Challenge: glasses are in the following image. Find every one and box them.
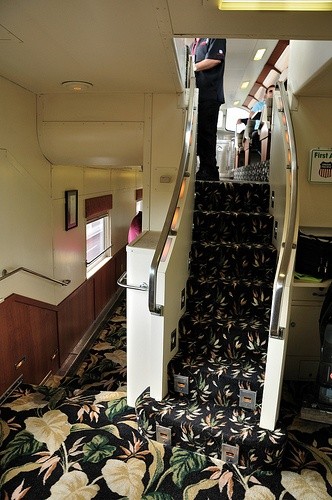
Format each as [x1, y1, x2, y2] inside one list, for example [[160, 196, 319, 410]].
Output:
[[267, 90, 272, 93]]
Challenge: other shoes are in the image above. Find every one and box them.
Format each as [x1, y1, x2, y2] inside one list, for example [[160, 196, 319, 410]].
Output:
[[196, 167, 219, 181]]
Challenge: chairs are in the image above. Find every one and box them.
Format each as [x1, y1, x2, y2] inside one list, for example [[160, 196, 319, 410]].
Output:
[[235, 99, 272, 169]]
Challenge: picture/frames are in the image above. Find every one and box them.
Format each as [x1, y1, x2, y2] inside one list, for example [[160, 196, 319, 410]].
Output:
[[64, 189, 80, 231]]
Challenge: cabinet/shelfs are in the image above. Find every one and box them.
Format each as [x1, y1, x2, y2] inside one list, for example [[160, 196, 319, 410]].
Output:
[[287, 280, 331, 381]]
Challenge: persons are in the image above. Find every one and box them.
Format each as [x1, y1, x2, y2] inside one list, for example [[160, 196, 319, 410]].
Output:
[[265, 85, 276, 101], [189, 37, 226, 181]]
